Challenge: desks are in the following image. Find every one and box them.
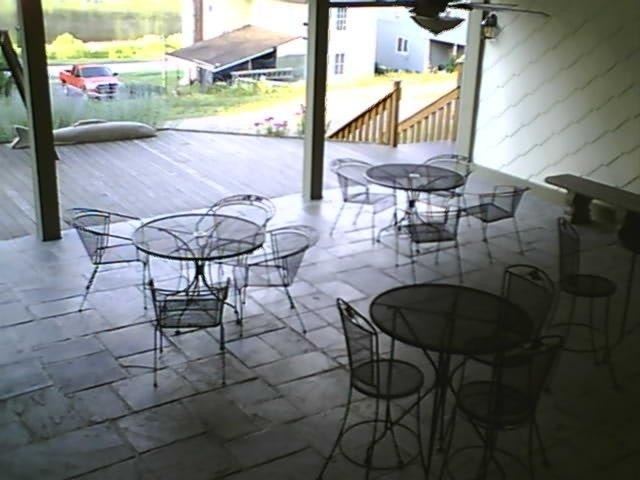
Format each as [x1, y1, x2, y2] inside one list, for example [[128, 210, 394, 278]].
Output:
[[132, 212, 266, 337], [370, 282, 536, 468], [366, 164, 465, 241]]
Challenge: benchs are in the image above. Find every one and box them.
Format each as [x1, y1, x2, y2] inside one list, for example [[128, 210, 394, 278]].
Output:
[[544, 173, 640, 252]]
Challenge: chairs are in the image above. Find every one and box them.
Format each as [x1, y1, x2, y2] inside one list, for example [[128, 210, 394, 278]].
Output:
[[318, 297, 430, 480], [194, 193, 272, 305], [416, 153, 475, 230], [235, 224, 319, 337], [620, 213, 640, 340], [545, 216, 620, 390], [454, 185, 531, 266], [330, 158, 401, 243], [396, 192, 462, 286], [465, 265, 556, 414], [63, 206, 155, 314], [147, 275, 230, 386], [442, 335, 565, 480]]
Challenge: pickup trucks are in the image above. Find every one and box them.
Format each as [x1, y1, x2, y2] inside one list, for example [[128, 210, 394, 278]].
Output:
[[59, 64, 123, 102]]
